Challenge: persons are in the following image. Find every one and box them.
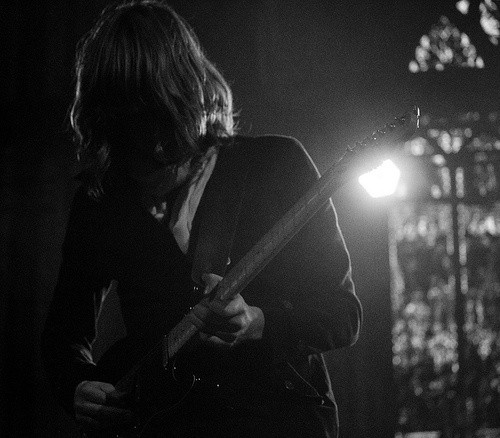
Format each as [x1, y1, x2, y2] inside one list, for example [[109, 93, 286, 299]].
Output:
[[40, 0, 364, 438]]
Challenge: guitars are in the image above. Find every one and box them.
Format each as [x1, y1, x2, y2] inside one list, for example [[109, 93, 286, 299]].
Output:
[[96, 104, 420, 438]]
